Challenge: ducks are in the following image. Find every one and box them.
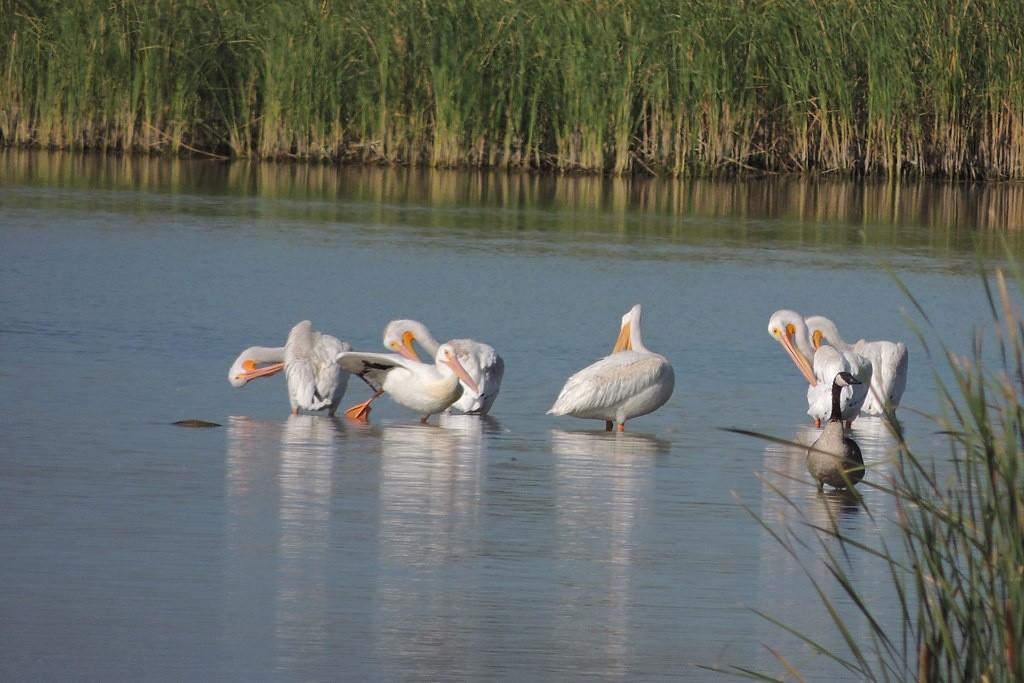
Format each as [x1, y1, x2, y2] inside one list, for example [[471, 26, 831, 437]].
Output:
[[806, 371, 865, 493]]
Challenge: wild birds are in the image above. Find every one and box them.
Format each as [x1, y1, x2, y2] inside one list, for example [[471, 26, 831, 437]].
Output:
[[335, 343, 479, 427], [228, 319, 354, 418], [805, 315, 909, 416], [767, 310, 873, 429], [380, 319, 506, 416], [544, 303, 676, 433]]
